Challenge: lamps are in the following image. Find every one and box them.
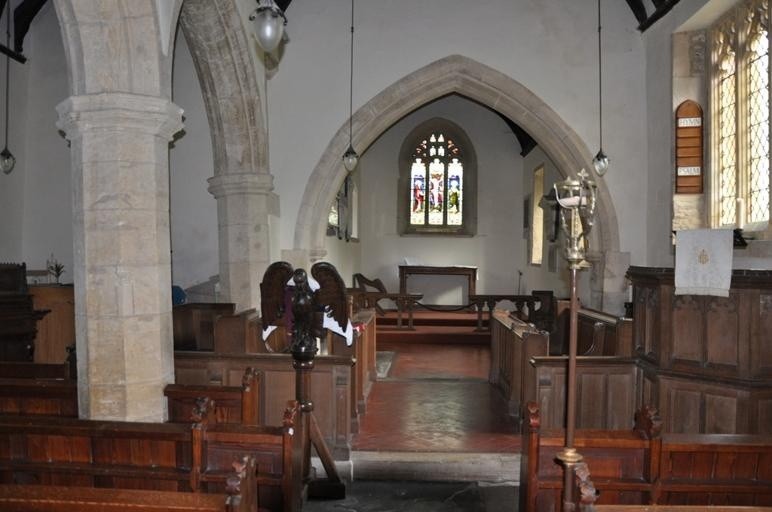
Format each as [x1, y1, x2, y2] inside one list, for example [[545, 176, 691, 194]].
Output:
[[249, 0, 288, 53], [342, 0, 359, 172], [592, 0, 610, 177], [0, 0, 14, 173]]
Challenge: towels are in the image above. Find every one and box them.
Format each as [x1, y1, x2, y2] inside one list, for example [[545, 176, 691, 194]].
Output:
[[674, 229, 734, 298]]
